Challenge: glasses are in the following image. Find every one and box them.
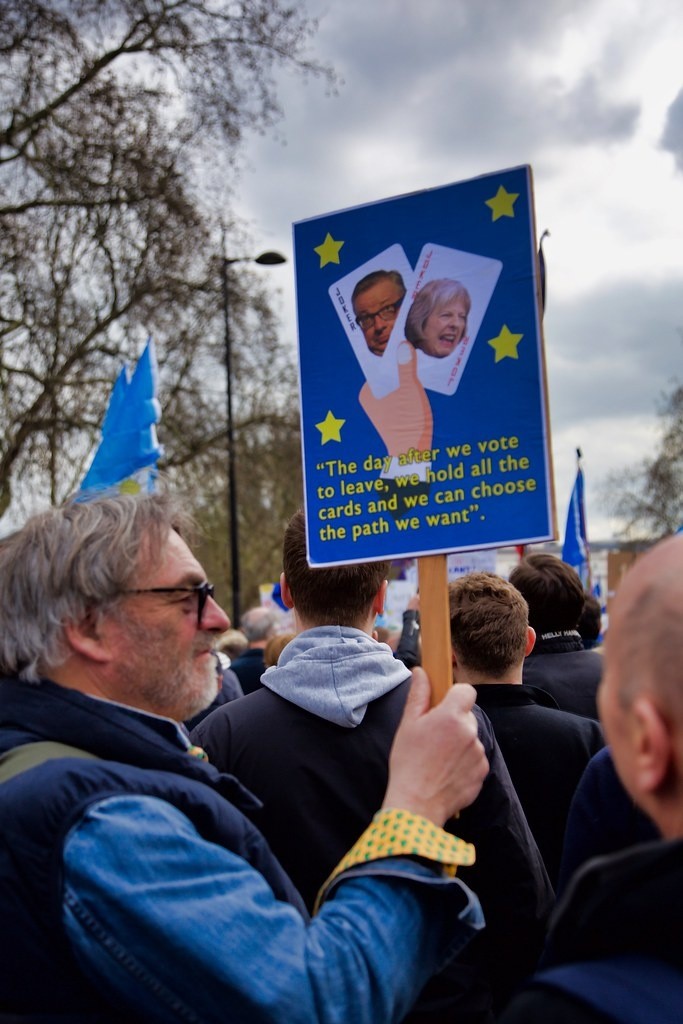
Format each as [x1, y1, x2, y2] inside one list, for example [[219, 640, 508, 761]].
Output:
[[355, 296, 405, 329], [120, 581, 215, 628]]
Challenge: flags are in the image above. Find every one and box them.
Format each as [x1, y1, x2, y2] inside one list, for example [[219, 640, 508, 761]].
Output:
[[69, 340, 161, 502], [560, 461, 602, 612]]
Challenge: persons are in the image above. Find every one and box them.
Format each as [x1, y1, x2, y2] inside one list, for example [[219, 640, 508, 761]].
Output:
[[0, 500, 683, 1023]]
